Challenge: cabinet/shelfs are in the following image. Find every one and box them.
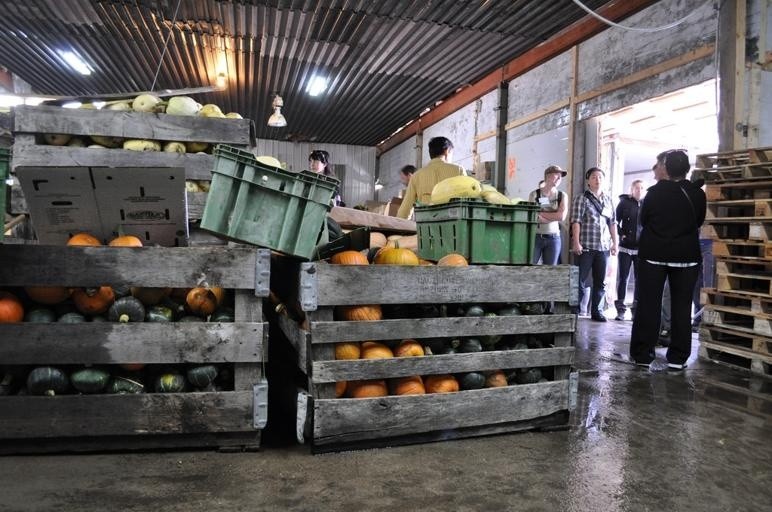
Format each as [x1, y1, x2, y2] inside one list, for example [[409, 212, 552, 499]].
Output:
[[694, 146, 772, 381]]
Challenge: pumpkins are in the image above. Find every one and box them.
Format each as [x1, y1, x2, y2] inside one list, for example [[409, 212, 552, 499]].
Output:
[[302, 242, 556, 398], [431, 174, 536, 204], [0, 221, 235, 396], [38, 93, 283, 192]]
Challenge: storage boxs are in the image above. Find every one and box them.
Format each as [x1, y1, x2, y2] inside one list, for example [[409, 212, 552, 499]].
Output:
[[413, 198, 540, 267], [10, 103, 258, 246], [0, 241, 271, 453], [200, 144, 340, 260], [278, 262, 579, 452]]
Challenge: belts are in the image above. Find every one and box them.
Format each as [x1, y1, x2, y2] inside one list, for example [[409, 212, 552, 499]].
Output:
[[537, 234, 557, 239]]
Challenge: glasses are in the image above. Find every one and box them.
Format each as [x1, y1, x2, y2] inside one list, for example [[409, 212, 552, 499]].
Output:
[[312, 149, 326, 163]]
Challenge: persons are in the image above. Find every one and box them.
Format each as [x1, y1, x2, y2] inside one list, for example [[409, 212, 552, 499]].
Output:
[[529, 165, 569, 313], [309, 151, 342, 206], [652, 150, 672, 347], [691, 155, 713, 334], [396, 137, 468, 220], [630, 152, 706, 370], [614, 179, 645, 322], [569, 168, 618, 321], [400, 164, 417, 185]]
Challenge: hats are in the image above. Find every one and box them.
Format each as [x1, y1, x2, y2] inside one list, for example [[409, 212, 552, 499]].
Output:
[[545, 165, 567, 176]]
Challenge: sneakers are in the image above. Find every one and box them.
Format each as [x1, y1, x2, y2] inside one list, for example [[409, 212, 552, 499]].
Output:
[[592, 311, 606, 321], [616, 312, 624, 320], [668, 362, 687, 369], [629, 356, 649, 366]]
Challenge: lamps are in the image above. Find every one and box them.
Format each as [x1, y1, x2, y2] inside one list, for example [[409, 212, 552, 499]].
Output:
[[267, 95, 288, 128]]
